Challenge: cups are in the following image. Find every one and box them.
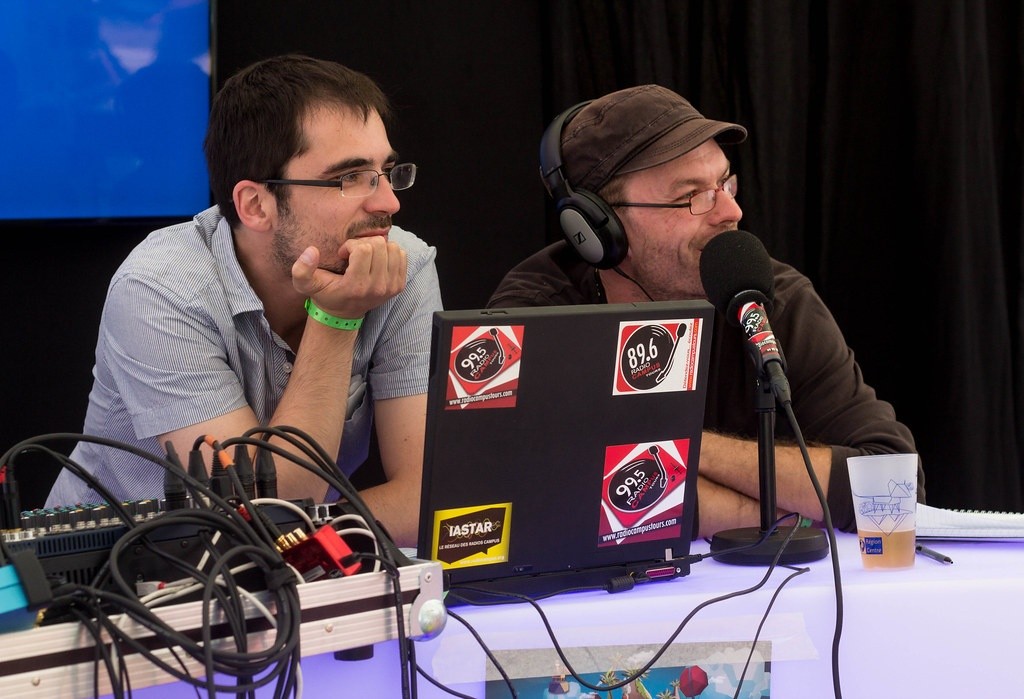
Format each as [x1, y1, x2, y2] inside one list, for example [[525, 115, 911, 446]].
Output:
[[846, 453, 918, 571]]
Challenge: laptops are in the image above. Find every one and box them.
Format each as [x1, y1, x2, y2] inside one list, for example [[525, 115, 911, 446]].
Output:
[[415, 298, 714, 608]]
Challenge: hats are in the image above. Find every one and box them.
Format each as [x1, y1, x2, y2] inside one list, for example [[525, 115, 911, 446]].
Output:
[[540, 84, 748, 201]]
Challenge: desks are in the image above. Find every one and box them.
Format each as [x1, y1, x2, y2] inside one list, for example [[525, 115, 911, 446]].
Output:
[[135, 530, 1024, 699]]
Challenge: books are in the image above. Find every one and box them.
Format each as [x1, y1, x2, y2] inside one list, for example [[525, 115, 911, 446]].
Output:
[[912, 501, 1024, 543]]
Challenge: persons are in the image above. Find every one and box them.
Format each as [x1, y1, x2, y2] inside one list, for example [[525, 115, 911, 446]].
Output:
[[485, 84, 928, 542], [43, 57, 444, 548]]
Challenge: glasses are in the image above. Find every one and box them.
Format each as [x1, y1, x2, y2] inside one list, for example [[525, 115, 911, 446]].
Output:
[[258, 163, 417, 198], [607, 173, 739, 216]]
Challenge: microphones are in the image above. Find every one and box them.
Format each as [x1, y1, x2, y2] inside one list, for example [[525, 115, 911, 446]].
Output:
[[699, 230, 793, 404]]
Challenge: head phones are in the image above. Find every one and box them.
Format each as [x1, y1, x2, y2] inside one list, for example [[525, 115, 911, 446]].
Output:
[[538, 99, 629, 271]]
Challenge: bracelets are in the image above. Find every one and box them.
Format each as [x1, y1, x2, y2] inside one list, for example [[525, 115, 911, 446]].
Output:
[[800, 516, 813, 528], [303, 298, 364, 331]]
